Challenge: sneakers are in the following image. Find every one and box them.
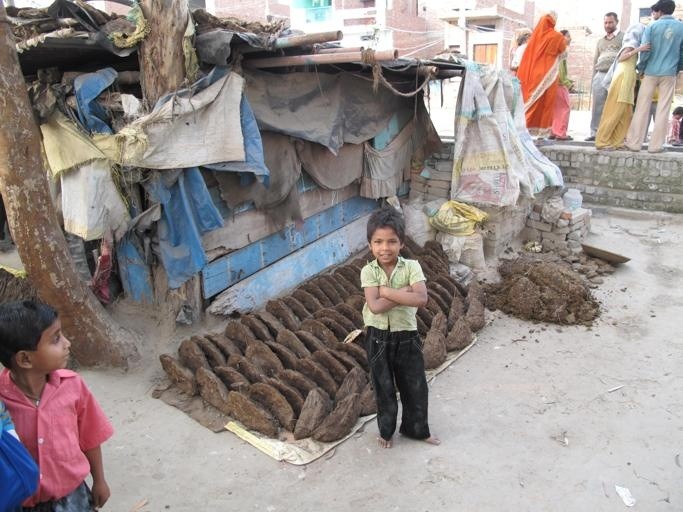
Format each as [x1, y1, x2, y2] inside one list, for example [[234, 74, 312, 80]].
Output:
[[547, 135, 555, 140], [555, 136, 573, 141]]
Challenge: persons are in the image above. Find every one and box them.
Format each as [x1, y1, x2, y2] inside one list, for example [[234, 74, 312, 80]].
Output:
[[650, 5, 660, 20], [361, 207, 441, 449], [0, 398, 40, 507], [640, 74, 675, 141], [668, 106, 682, 145], [549, 30, 573, 141], [0, 299, 114, 508], [584, 12, 625, 141], [625, 0, 683, 154], [597, 21, 650, 150], [517, 9, 568, 140]]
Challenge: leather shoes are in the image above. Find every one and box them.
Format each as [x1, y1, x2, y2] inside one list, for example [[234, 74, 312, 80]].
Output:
[[584, 136, 595, 141]]
